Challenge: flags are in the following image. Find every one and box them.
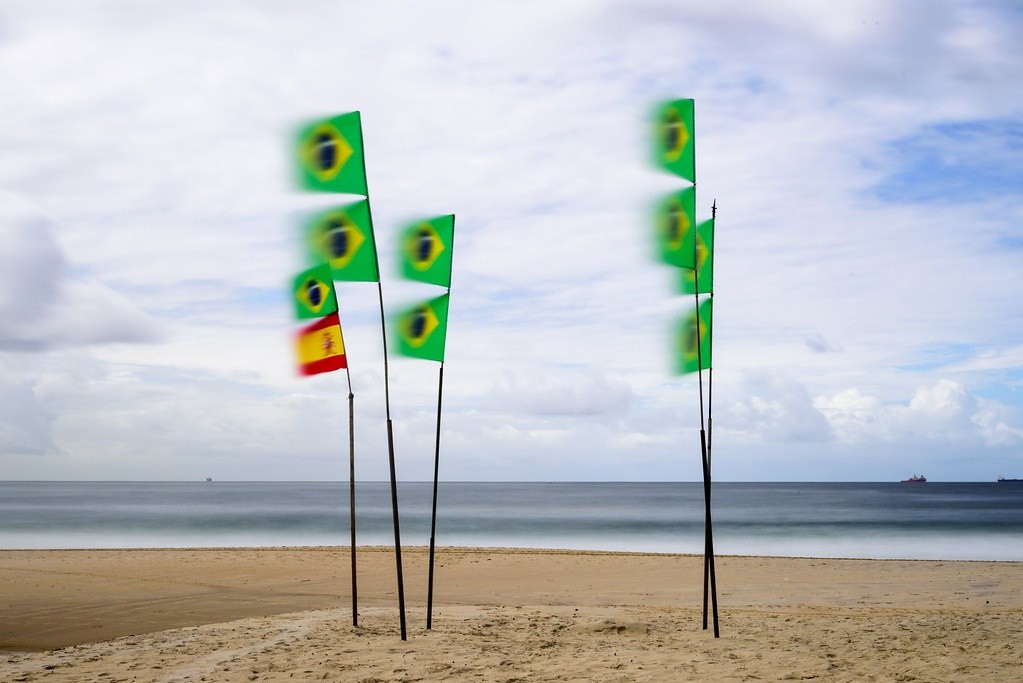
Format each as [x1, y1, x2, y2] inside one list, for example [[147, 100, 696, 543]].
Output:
[[294, 314, 347, 376], [292, 262, 336, 320], [306, 199, 378, 286], [652, 185, 693, 270], [654, 99, 695, 184], [672, 298, 711, 376], [295, 111, 367, 197], [676, 218, 713, 295], [390, 293, 452, 363], [397, 214, 452, 288]]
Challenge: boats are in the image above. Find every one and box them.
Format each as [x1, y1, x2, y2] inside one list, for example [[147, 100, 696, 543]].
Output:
[[998, 478, 1023, 483], [901, 475, 926, 482]]
